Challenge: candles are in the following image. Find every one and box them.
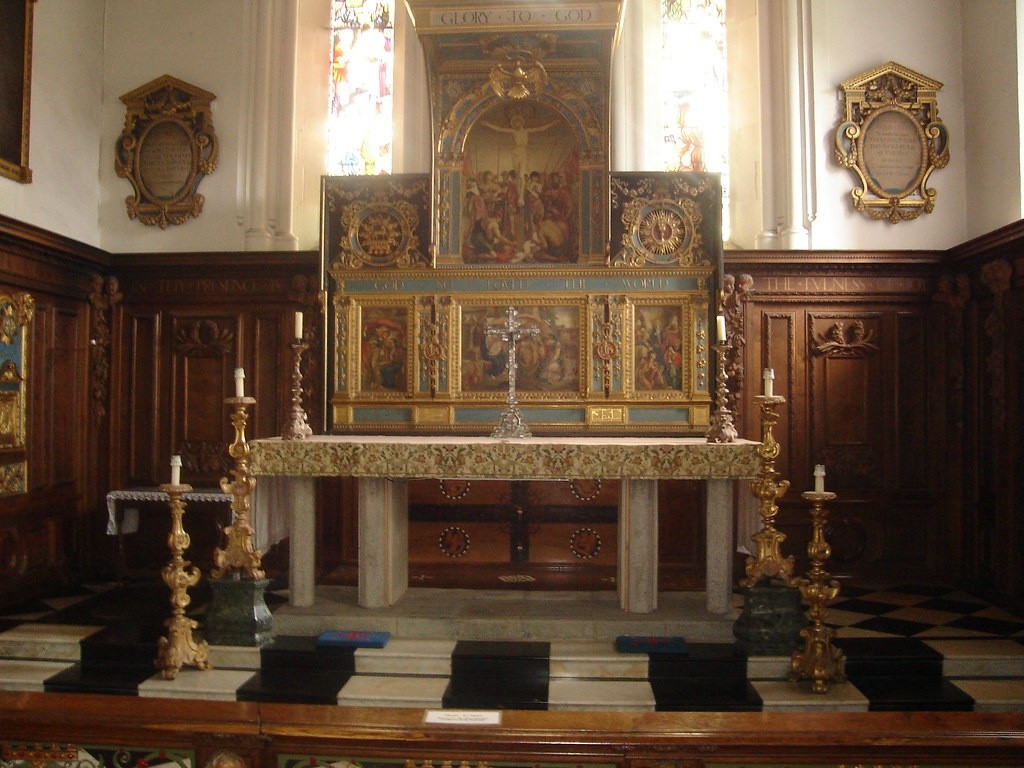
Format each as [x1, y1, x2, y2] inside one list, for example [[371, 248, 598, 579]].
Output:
[[234, 368, 246, 397], [716, 315, 727, 344], [813, 462, 826, 493], [171, 455, 182, 485], [763, 366, 775, 395], [295, 312, 303, 339]]
